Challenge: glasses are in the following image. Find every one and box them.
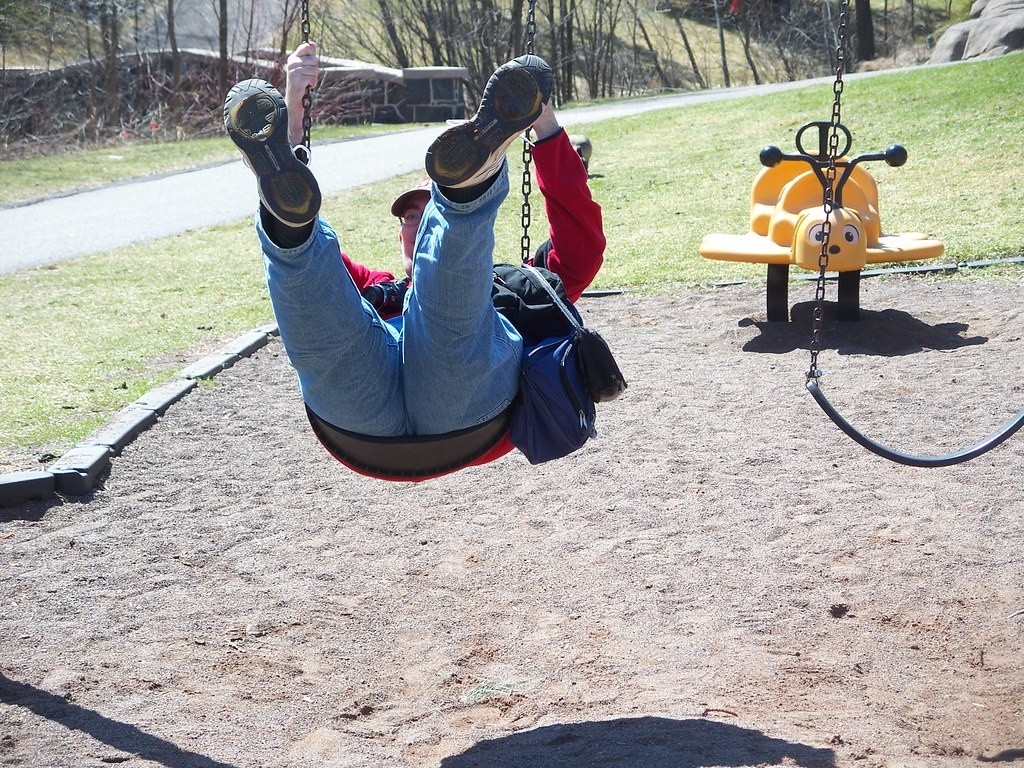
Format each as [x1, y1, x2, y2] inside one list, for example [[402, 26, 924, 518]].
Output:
[[399, 214, 423, 226]]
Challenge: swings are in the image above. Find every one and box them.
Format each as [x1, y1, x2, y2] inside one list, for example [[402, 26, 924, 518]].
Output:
[[296, 0, 539, 478], [804, 2, 1024, 474]]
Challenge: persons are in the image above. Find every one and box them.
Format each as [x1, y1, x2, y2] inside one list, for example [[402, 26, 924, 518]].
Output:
[[223, 42, 606, 482]]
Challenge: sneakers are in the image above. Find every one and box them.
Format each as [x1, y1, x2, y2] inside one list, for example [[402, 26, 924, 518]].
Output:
[[425, 54, 553, 189], [223, 78, 322, 227]]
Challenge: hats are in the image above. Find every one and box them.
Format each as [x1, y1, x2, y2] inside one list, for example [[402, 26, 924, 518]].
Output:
[[391, 178, 433, 216]]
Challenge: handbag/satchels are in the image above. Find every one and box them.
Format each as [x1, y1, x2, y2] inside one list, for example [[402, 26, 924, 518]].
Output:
[[575, 330, 629, 402], [510, 335, 596, 464]]
